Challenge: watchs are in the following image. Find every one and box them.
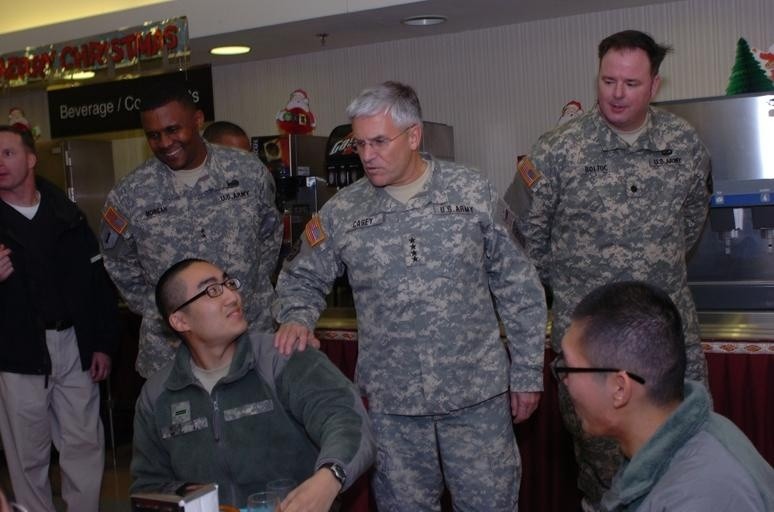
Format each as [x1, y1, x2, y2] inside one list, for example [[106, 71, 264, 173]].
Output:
[[319, 462, 347, 488]]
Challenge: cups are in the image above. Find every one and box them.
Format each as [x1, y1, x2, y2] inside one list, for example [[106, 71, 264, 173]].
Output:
[[247, 478, 298, 512]]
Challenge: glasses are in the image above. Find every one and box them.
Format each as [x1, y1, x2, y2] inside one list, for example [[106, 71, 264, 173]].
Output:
[[351, 123, 416, 154], [172, 277, 242, 314], [551, 350, 646, 384]]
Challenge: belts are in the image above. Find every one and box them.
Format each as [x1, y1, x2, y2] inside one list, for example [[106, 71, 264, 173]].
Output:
[[44, 321, 74, 332]]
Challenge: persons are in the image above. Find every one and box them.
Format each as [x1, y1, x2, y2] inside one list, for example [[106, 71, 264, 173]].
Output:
[[202, 120, 250, 151], [0, 125, 119, 512], [125, 257, 375, 512], [504, 29, 713, 512], [559, 281, 774, 512], [273, 79, 550, 512], [96, 83, 286, 380]]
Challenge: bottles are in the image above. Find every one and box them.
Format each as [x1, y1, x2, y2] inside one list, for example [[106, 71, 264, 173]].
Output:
[[276, 167, 296, 205]]
